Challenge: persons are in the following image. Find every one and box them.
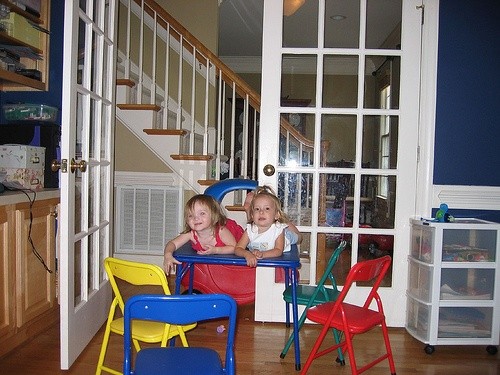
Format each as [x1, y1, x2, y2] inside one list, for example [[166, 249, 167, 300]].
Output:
[[162, 194, 236, 276], [235, 185, 302, 268]]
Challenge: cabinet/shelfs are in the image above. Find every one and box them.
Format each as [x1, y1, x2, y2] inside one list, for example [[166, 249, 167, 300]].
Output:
[[405, 217, 500, 357], [0, 188, 61, 357], [0, 0, 49, 92]]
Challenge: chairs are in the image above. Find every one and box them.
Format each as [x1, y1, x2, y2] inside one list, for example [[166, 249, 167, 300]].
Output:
[[96, 257, 197, 375], [322, 159, 374, 228], [168, 178, 302, 372], [300, 255, 396, 375], [278, 240, 345, 366], [122, 293, 237, 375]]
